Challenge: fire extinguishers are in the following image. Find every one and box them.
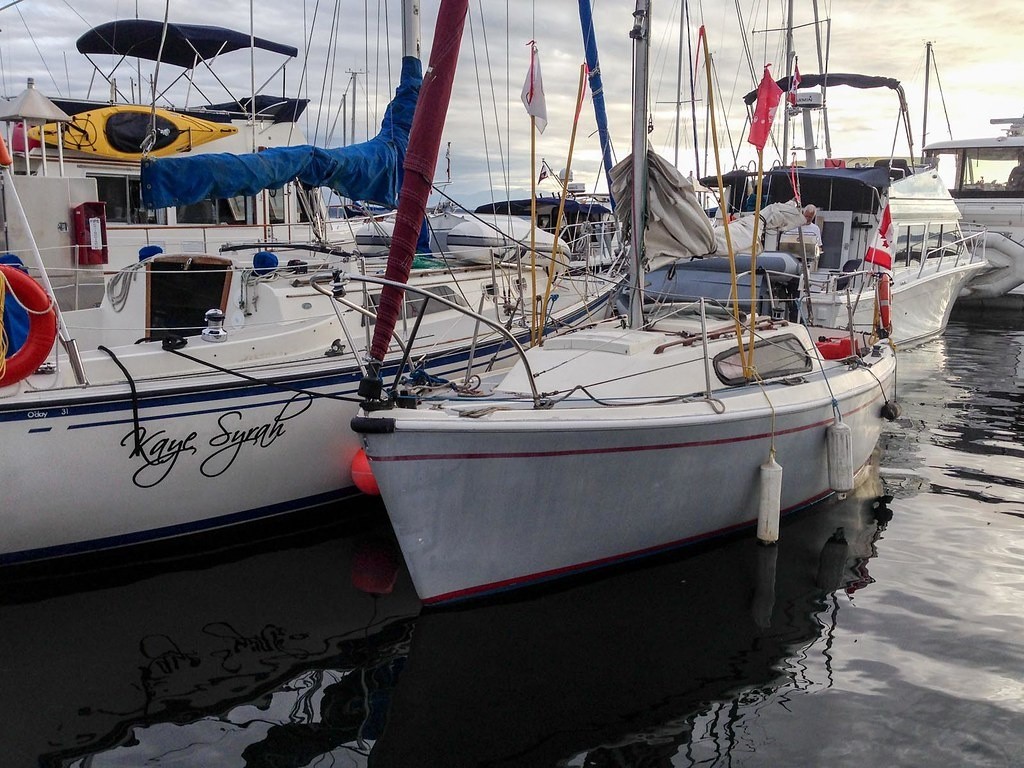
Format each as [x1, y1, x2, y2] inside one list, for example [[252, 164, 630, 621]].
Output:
[[85, 209, 103, 264]]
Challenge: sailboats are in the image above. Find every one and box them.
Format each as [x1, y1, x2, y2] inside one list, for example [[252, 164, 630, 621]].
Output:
[[1, 0, 1023, 615]]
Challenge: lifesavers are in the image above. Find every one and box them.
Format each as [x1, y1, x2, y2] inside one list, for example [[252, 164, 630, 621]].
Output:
[[0, 263, 56, 388], [881, 274, 890, 331]]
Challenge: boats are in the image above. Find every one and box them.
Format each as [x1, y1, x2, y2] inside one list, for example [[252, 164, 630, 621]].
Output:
[[24, 104, 240, 163], [353, 210, 573, 278]]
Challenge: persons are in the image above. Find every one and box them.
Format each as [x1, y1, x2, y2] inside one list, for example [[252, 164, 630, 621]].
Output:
[[786, 204, 824, 254], [1008, 152, 1024, 191]]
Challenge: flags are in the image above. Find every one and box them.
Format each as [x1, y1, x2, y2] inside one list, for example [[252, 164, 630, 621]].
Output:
[[538, 165, 548, 185], [748, 69, 783, 152], [521, 50, 548, 135], [786, 64, 801, 106], [864, 198, 895, 271]]
